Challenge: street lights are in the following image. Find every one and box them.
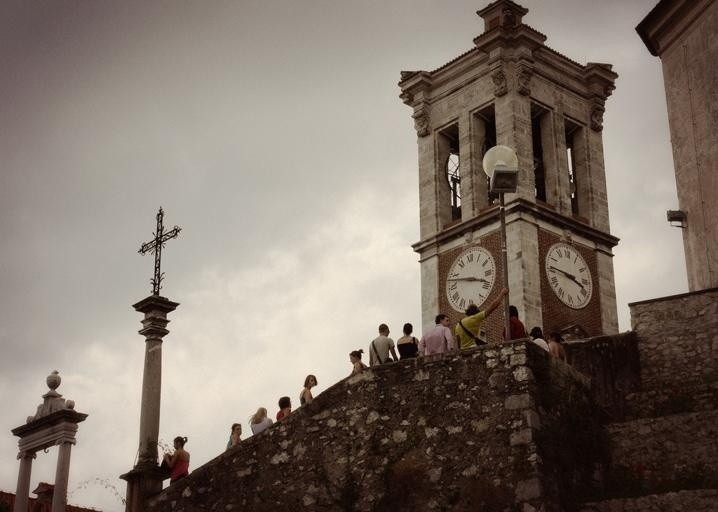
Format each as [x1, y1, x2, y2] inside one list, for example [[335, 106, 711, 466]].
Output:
[[478, 143, 522, 344]]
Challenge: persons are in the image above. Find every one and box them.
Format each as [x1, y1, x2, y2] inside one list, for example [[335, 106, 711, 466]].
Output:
[[529, 324, 551, 355], [348, 348, 366, 373], [417, 314, 455, 358], [162, 435, 192, 484], [547, 331, 567, 364], [225, 422, 243, 451], [454, 287, 510, 350], [396, 323, 419, 359], [502, 304, 525, 341], [367, 323, 399, 366], [248, 407, 273, 435], [298, 374, 318, 406], [275, 395, 292, 421]]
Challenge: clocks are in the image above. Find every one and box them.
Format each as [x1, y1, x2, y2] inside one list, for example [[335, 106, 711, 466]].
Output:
[[545, 241, 593, 311], [445, 246, 496, 314]]
